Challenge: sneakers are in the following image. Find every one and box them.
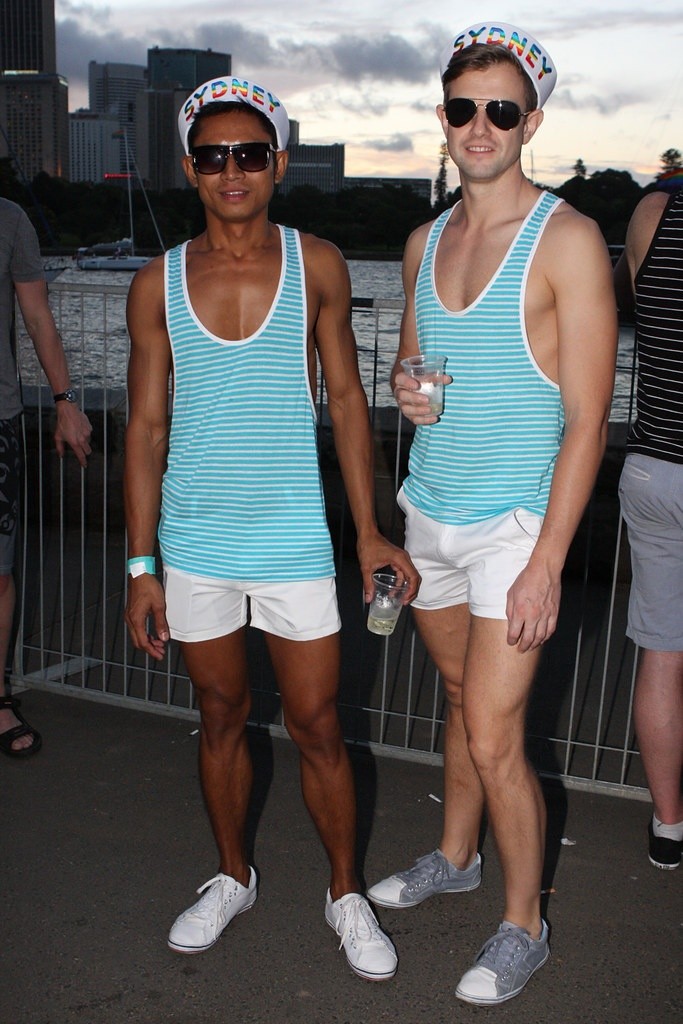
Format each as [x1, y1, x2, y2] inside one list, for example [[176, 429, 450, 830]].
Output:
[[649, 820, 683, 870], [169, 867, 258, 953], [368, 850, 482, 909], [324, 887, 399, 982], [455, 920, 551, 1006]]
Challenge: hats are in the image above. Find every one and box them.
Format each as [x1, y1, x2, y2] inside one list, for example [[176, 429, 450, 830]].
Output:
[[177, 77, 290, 158], [439, 22, 557, 108]]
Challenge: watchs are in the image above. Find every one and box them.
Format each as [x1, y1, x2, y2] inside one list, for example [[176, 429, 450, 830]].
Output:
[[54, 388, 78, 404]]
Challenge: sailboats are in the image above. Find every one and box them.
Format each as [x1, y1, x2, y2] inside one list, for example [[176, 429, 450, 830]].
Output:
[[77, 127, 166, 272], [0, 123, 72, 284]]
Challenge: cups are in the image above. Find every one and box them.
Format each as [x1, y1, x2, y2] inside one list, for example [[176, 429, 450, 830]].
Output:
[[400, 355, 446, 416], [367, 573, 410, 635]]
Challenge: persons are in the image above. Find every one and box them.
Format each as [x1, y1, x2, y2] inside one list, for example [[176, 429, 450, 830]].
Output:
[[0, 197, 93, 757], [126, 76, 423, 979], [366, 21, 618, 1005], [626, 187, 683, 870]]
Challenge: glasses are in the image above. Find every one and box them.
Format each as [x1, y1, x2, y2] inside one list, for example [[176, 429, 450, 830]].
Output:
[[191, 143, 275, 176], [443, 98, 532, 132]]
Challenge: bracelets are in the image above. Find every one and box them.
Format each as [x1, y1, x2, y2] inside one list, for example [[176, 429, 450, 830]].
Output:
[[125, 556, 156, 578]]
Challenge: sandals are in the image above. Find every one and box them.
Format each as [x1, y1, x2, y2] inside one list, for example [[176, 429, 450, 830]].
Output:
[[0, 697, 42, 759]]
